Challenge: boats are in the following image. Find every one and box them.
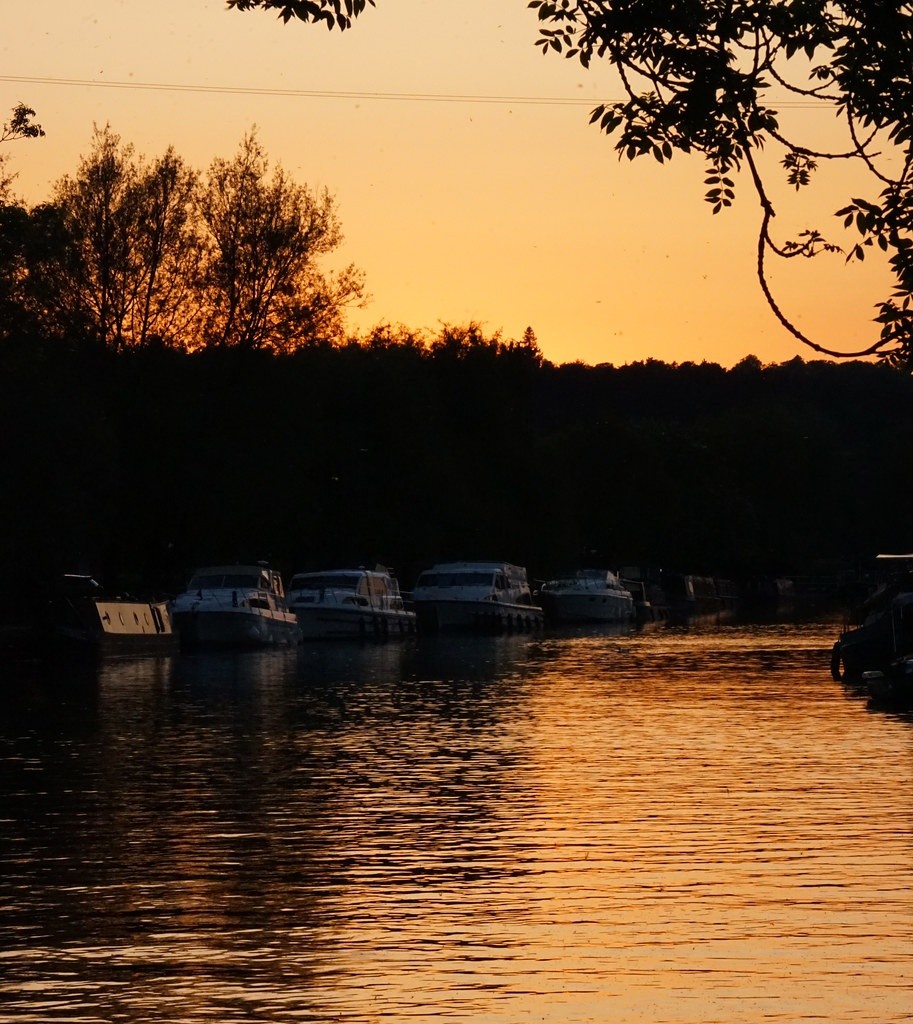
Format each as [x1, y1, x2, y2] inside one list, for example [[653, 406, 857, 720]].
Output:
[[41, 574, 173, 641], [830, 553, 913, 680], [541, 570, 637, 622], [412, 562, 544, 634], [285, 564, 417, 639], [173, 559, 304, 645], [649, 558, 841, 619]]
